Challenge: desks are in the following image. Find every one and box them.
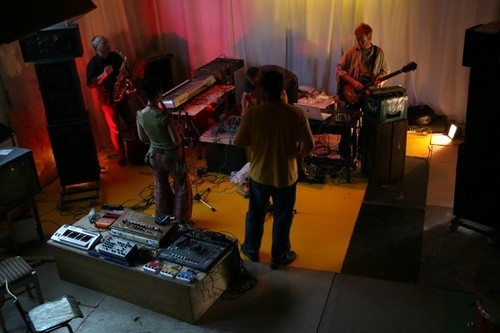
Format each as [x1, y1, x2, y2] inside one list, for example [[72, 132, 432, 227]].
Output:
[[308, 111, 358, 180], [0, 201, 45, 256], [56, 231, 239, 325], [199, 121, 246, 174]]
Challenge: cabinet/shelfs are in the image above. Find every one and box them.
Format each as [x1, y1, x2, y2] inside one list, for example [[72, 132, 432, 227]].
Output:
[[450, 24, 500, 243]]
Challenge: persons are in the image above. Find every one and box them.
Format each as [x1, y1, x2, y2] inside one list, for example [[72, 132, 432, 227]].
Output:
[[337, 23, 389, 172], [138, 72, 193, 226], [86, 35, 144, 166], [233, 65, 314, 270]]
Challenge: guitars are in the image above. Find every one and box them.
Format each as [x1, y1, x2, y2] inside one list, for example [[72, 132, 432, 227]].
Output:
[[337, 61, 418, 105]]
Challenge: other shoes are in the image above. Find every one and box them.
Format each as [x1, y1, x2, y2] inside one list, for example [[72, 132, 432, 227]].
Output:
[[240, 243, 260, 262], [270, 250, 296, 270], [117, 154, 127, 166]]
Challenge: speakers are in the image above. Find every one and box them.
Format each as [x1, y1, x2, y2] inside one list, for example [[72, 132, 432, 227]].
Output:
[[454, 67, 500, 232], [204, 143, 246, 174], [35, 61, 99, 183], [143, 56, 178, 95]]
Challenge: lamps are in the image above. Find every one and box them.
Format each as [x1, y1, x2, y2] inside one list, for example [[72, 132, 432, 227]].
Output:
[[448, 124, 462, 138]]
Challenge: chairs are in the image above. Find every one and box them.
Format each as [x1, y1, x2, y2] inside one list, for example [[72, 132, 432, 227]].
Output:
[[0, 255, 84, 333]]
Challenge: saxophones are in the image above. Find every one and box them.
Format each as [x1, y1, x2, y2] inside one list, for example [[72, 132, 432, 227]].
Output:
[[111, 48, 137, 102]]
